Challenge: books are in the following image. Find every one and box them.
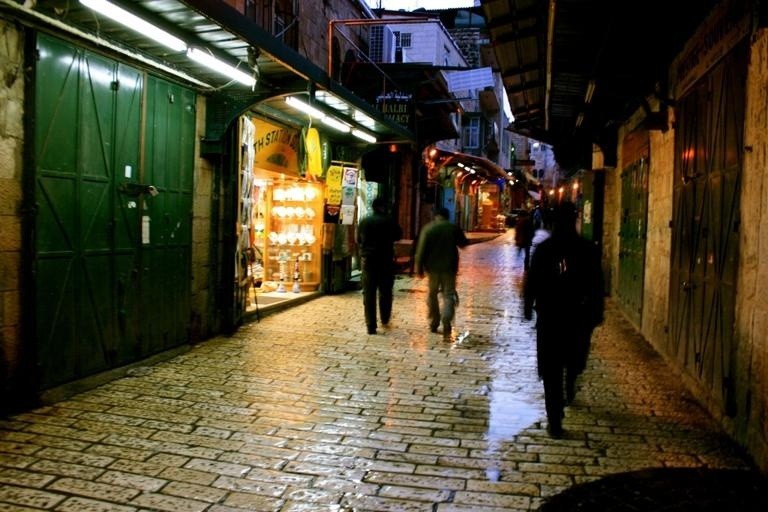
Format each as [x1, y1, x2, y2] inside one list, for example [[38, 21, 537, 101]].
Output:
[[323, 165, 359, 227]]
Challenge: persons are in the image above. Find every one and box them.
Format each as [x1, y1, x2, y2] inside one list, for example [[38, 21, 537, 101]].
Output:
[[511, 194, 562, 272], [415, 203, 467, 340], [354, 193, 404, 336], [521, 201, 605, 441], [428, 205, 459, 320]]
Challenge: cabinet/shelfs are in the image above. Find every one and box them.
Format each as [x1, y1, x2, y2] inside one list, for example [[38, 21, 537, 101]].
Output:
[[262, 185, 321, 291]]
